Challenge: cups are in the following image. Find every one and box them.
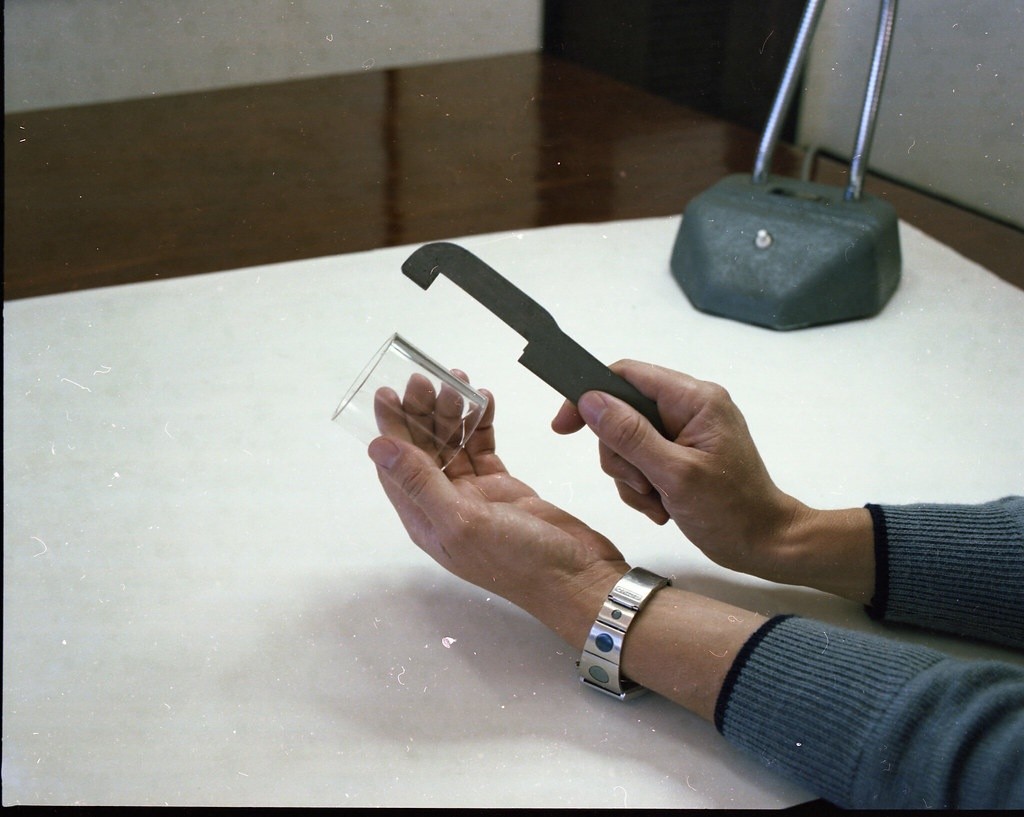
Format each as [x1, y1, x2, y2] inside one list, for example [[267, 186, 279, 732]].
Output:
[[330, 333, 490, 472]]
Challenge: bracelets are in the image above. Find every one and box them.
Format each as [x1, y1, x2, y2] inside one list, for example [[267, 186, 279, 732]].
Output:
[[579, 566, 672, 699]]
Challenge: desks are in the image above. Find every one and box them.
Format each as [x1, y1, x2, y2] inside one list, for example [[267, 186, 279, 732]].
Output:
[[2, 55, 1024, 816]]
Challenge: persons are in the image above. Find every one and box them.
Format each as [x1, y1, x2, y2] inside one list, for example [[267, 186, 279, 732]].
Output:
[[369, 358, 1023, 810]]
